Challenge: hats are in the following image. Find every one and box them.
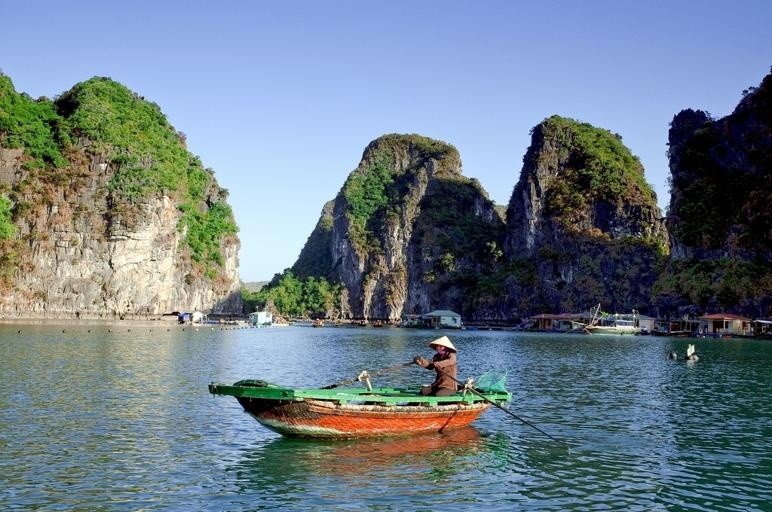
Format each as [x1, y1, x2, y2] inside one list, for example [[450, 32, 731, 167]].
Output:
[[429, 336, 457, 352]]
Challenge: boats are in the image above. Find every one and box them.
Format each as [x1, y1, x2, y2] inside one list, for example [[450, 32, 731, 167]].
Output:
[[570, 302, 643, 335], [286, 316, 401, 328], [666, 344, 702, 364], [199, 371, 523, 443]]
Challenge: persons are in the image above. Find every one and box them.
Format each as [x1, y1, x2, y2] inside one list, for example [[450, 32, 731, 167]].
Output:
[[414, 344, 458, 397]]
